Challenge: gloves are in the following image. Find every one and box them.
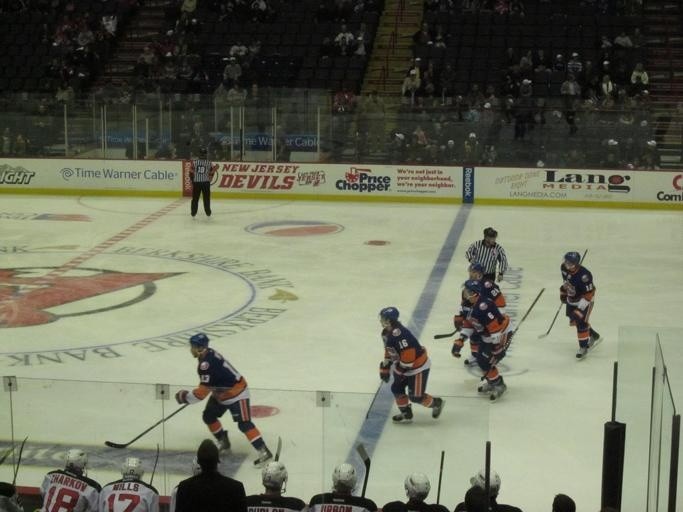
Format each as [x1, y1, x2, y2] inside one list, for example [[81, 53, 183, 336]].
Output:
[[392, 365, 405, 386], [175, 390, 188, 404], [492, 344, 505, 360], [379, 366, 390, 383], [569, 314, 579, 326], [451, 339, 463, 357], [454, 315, 464, 331], [559, 287, 566, 303]]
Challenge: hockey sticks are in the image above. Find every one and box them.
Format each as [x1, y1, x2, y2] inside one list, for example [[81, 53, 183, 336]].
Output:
[[356, 443, 370, 499], [434, 330, 458, 339], [0, 447, 17, 465], [149, 444, 159, 484], [537, 249, 588, 339], [105, 403, 188, 449], [465, 287, 545, 385], [11, 436, 27, 485], [274, 437, 282, 462]]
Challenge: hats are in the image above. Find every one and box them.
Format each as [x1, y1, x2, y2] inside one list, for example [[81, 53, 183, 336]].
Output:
[[197, 440, 219, 465]]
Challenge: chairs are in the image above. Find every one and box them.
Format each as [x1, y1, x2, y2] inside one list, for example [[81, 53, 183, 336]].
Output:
[[1, 2, 681, 173]]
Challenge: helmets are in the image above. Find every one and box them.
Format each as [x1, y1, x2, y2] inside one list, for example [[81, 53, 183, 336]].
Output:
[[465, 278, 486, 293], [483, 227, 498, 239], [469, 470, 500, 498], [65, 449, 88, 470], [331, 464, 357, 489], [120, 457, 144, 477], [189, 333, 208, 348], [191, 456, 201, 476], [403, 472, 429, 500], [468, 261, 483, 272], [380, 307, 399, 320], [261, 462, 288, 489], [564, 252, 579, 265]]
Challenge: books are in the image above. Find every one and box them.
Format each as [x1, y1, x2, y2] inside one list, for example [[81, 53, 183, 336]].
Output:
[[450, 227, 513, 400]]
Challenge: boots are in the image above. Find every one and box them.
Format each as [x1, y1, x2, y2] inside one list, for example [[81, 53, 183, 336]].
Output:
[[489, 376, 506, 400], [392, 404, 413, 421], [586, 327, 599, 347], [249, 438, 272, 465], [215, 430, 231, 451], [464, 357, 477, 364], [478, 382, 492, 392], [575, 340, 587, 358], [427, 398, 442, 418]]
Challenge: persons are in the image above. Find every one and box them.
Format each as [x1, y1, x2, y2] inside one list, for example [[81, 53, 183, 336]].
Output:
[[40, 449, 103, 512], [187, 145, 215, 217], [98, 456, 160, 512], [244, 461, 306, 512], [377, 307, 446, 424], [174, 333, 274, 468], [310, 464, 378, 512], [559, 250, 601, 359], [1, 1, 681, 170], [552, 494, 577, 512], [382, 472, 448, 512], [454, 470, 521, 512], [171, 440, 246, 511]]
